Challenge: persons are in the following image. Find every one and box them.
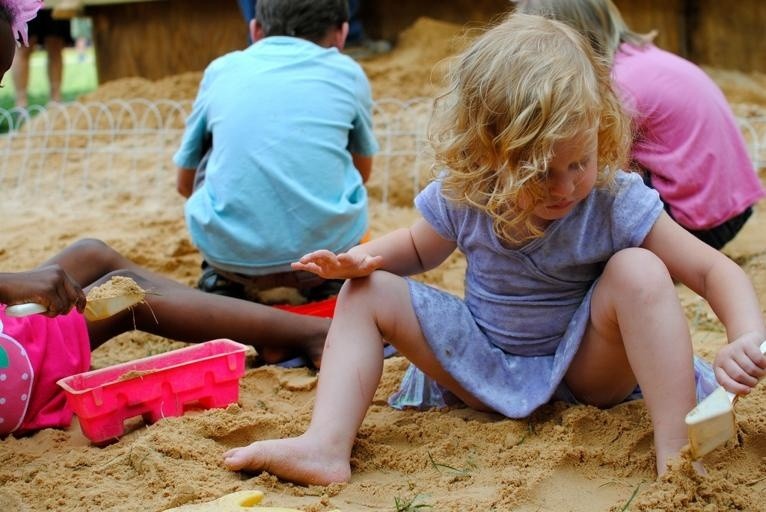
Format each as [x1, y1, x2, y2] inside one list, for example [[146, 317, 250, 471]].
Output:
[[10, 7, 76, 123], [170, 0, 381, 304], [511, 2, 764, 282], [0, 237, 396, 439], [224, 13, 766, 490]]
